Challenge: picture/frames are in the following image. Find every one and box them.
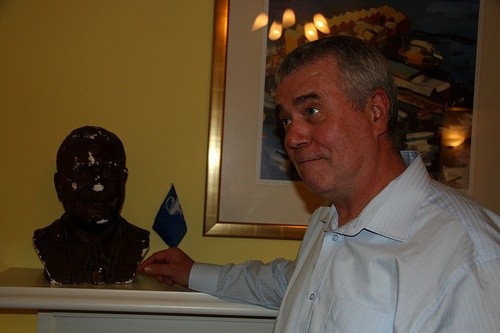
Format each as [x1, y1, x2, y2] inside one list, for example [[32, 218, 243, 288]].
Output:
[[198, 0, 500, 242]]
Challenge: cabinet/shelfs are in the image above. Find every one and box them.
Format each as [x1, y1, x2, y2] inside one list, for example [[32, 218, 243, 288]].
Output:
[[0, 266, 288, 333]]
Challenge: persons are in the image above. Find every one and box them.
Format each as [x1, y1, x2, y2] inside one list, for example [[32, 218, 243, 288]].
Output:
[[135, 35, 499, 333], [33, 125, 149, 285]]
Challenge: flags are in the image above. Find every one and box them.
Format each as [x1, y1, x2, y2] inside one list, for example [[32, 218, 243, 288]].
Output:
[[152, 184, 187, 248]]
